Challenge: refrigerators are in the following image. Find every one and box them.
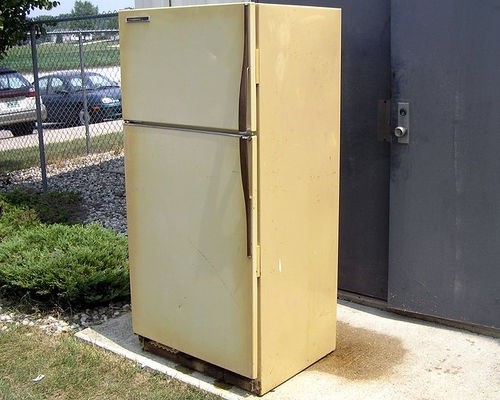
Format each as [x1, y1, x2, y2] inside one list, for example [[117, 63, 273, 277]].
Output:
[[116, 3, 342, 396]]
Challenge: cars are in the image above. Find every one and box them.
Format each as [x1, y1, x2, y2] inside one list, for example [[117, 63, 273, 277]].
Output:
[[32, 72, 122, 125], [0, 68, 47, 137]]
